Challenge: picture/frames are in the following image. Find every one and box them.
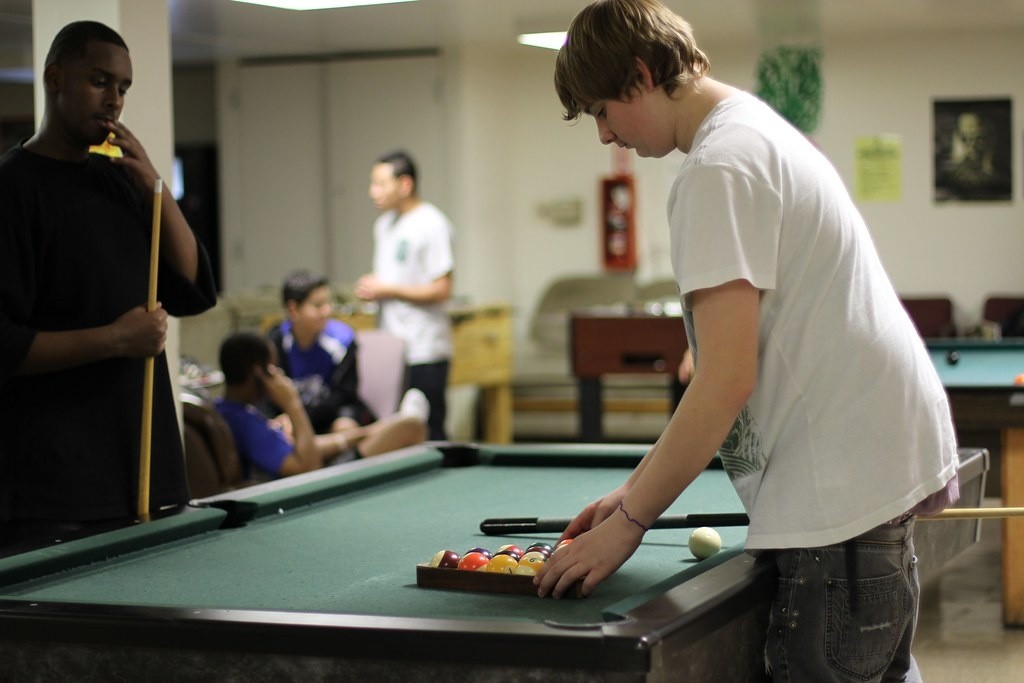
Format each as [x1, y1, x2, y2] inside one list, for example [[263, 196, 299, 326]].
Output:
[[932, 97, 1013, 205]]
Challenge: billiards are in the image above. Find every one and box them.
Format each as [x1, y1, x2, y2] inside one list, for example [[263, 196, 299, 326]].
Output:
[[1013, 372, 1022, 384], [944, 347, 959, 367], [427, 531, 578, 589], [686, 524, 724, 562]]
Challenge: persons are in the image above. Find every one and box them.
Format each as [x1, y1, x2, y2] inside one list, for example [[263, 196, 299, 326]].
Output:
[[208, 148, 454, 482], [0, 22, 216, 546], [531, 1, 959, 683]]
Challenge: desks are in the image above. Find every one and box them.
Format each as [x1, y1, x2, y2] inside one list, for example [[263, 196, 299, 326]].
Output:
[[0, 439, 771, 683], [924, 336, 1024, 628], [266, 302, 513, 445]]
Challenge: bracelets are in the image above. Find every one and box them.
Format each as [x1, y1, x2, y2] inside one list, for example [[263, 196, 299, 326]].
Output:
[[619, 500, 649, 532]]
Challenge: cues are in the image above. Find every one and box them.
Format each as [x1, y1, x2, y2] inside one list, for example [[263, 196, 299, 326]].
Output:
[[133, 172, 171, 527], [479, 505, 1024, 535]]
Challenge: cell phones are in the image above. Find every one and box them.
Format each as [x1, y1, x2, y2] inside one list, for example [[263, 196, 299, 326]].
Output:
[[255, 369, 277, 388]]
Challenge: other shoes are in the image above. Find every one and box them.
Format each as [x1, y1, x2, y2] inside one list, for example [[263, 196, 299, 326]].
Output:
[[399, 388, 432, 423]]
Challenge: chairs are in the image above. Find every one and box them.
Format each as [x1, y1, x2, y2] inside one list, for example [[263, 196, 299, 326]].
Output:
[[568, 316, 689, 444], [900, 295, 959, 337], [172, 391, 259, 499], [981, 293, 1024, 338]]
